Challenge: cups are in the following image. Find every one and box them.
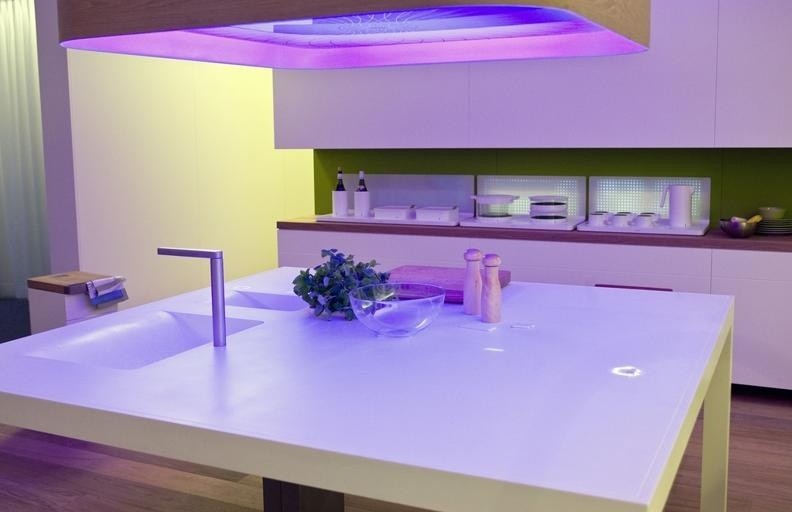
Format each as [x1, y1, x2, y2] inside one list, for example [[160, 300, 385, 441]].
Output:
[[589, 210, 658, 227]]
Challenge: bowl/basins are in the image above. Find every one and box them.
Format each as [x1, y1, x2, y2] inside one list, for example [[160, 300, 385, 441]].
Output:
[[719, 218, 758, 239], [759, 206, 787, 219], [350, 283, 447, 339]]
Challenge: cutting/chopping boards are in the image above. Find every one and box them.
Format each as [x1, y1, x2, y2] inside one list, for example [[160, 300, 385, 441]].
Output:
[[383, 264, 511, 305]]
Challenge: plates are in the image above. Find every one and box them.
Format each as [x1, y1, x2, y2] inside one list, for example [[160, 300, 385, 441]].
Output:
[[756, 219, 791, 236]]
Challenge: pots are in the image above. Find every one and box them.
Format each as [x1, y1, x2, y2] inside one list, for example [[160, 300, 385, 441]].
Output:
[[471, 193, 519, 222]]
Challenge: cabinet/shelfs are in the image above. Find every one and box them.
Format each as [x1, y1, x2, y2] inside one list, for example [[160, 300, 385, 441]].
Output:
[[710, 238, 792, 401], [469, 0, 713, 148], [273, 63, 469, 150], [714, 0, 792, 149], [277, 223, 471, 268], [472, 230, 710, 295]]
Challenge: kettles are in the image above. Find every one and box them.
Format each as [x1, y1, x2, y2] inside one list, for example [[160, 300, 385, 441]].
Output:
[[660, 185, 695, 229]]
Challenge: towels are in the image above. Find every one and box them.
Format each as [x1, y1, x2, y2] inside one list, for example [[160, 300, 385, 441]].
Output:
[[87, 276, 129, 310]]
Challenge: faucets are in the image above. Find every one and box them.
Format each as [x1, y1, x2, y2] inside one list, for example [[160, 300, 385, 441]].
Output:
[[156, 247, 228, 348]]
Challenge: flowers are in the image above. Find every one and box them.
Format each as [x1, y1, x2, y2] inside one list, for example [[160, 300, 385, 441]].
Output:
[[292, 247, 399, 323]]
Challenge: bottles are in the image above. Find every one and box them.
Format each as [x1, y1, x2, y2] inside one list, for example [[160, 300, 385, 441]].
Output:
[[355, 170, 370, 219], [479, 254, 502, 323], [331, 167, 348, 217], [462, 248, 483, 315]]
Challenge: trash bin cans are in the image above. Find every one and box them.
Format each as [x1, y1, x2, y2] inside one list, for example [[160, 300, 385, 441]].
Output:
[[27, 270, 126, 335]]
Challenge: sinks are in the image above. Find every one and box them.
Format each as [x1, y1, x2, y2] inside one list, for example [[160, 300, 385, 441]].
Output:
[[202, 287, 322, 311], [19, 308, 264, 372]]
